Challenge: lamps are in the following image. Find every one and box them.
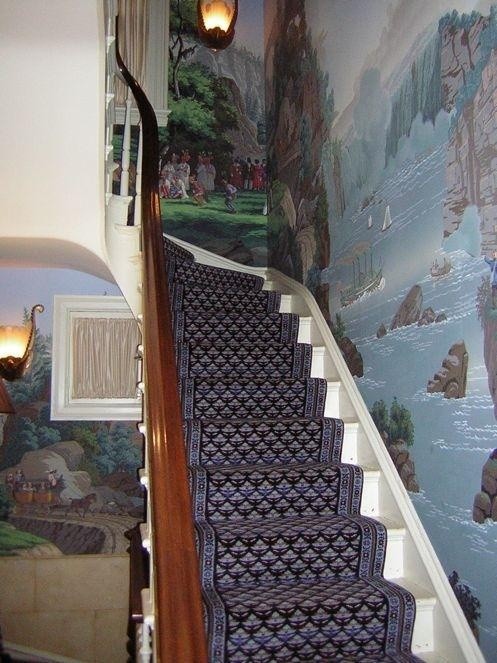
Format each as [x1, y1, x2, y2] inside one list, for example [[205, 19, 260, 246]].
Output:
[[0, 304, 45, 414], [197, 0, 239, 53]]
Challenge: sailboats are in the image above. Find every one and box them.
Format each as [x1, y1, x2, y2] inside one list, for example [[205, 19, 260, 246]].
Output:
[[368, 205, 393, 232], [339, 251, 383, 306]]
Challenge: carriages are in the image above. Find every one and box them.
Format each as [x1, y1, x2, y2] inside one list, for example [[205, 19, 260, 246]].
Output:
[[6, 472, 97, 521]]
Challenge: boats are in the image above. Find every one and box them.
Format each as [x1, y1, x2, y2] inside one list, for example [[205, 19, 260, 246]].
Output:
[[429, 257, 450, 276]]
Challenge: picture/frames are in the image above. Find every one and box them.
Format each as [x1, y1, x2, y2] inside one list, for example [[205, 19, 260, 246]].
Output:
[[49, 293, 142, 422], [103, 0, 171, 128]]
